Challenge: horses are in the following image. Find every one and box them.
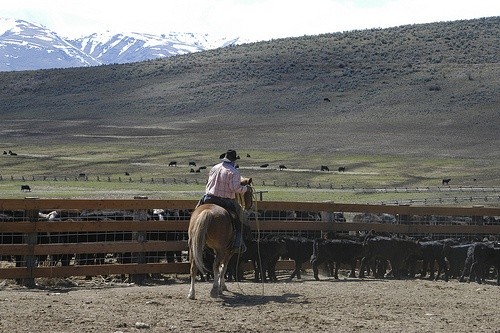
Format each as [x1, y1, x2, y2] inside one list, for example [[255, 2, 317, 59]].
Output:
[[20, 184, 31, 192], [188, 161, 197, 167], [337, 166, 346, 172], [320, 165, 330, 172], [323, 97, 331, 102], [246, 153, 251, 157], [442, 178, 452, 185], [124, 171, 130, 176], [0, 196, 500, 289], [189, 168, 195, 173], [3, 149, 7, 155], [196, 168, 200, 173], [278, 164, 288, 171], [78, 173, 86, 178], [199, 166, 207, 170], [184, 175, 255, 300], [169, 160, 177, 167], [260, 163, 270, 168], [8, 150, 17, 156]]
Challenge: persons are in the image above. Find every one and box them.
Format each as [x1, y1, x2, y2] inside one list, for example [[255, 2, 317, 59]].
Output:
[[205, 149, 250, 232]]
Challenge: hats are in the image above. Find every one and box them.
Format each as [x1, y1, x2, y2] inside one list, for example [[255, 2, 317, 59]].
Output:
[[219, 149, 240, 163]]
